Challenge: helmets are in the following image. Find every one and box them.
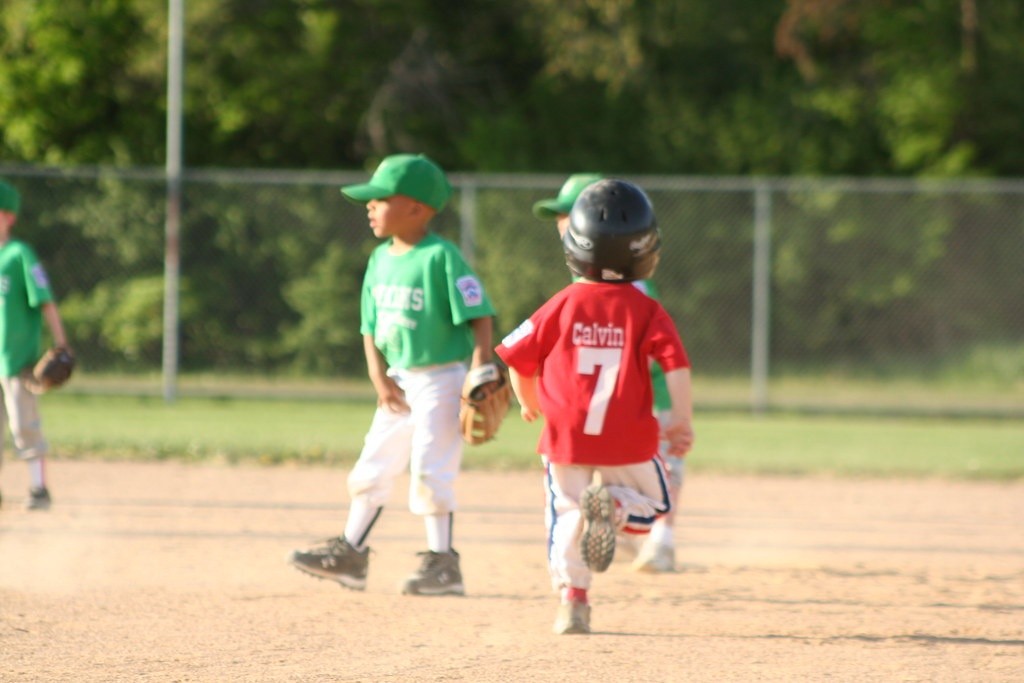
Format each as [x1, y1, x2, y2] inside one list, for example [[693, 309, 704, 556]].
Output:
[[563, 180, 662, 283]]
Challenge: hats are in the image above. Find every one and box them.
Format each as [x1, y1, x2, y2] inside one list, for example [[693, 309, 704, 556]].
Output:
[[0, 179, 21, 212], [533, 173, 602, 223], [339, 154, 448, 215]]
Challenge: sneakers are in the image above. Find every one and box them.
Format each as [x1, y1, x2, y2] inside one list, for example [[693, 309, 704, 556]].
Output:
[[579, 484, 615, 573], [552, 598, 593, 635], [291, 537, 371, 590], [403, 549, 465, 594]]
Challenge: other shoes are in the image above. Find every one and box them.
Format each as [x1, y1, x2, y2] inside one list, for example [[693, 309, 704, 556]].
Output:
[[26, 486, 49, 510], [614, 534, 636, 561], [635, 544, 676, 572]]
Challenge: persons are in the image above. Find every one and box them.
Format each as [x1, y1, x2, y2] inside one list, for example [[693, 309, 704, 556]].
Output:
[[532, 175, 686, 573], [290, 153, 500, 596], [495, 182, 694, 633], [0, 179, 74, 510]]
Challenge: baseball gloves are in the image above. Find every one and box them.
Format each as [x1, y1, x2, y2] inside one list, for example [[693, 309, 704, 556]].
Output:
[[457, 362, 512, 446], [20, 344, 77, 395]]
